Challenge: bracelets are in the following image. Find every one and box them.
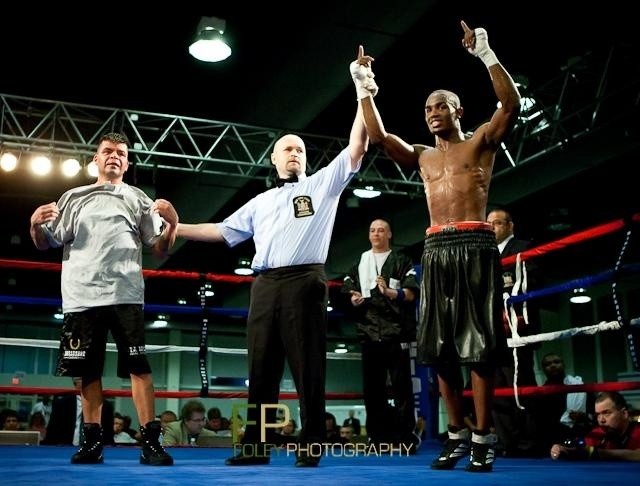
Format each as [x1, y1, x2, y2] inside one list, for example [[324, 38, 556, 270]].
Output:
[[392, 288, 406, 303]]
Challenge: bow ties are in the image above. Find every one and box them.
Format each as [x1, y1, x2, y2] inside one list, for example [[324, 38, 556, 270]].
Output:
[[276, 175, 298, 188]]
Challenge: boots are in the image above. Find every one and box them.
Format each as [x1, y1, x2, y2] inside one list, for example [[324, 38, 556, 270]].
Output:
[[430, 424, 473, 470], [72, 423, 104, 464], [140, 420, 173, 467], [464, 429, 498, 473]]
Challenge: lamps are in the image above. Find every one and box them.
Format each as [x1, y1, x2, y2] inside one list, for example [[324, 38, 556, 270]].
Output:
[[186, 12, 235, 67]]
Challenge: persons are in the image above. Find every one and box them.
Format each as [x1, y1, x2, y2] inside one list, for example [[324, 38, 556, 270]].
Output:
[[484, 205, 545, 458], [157, 42, 380, 472], [0, 353, 640, 468], [335, 218, 425, 454], [30, 131, 180, 468], [345, 14, 521, 474]]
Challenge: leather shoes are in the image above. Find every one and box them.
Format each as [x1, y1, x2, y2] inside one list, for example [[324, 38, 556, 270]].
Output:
[[224, 444, 272, 467], [294, 452, 321, 467], [364, 436, 416, 455]]
